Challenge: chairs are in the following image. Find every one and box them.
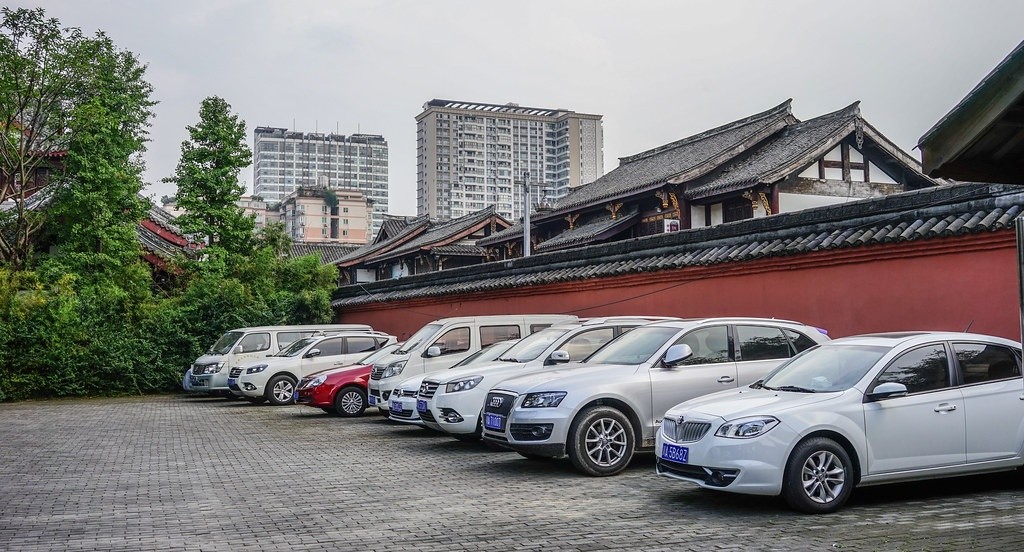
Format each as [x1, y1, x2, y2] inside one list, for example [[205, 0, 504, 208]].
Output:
[[706, 333, 726, 361], [680, 335, 700, 357], [457, 328, 468, 350], [568, 339, 589, 358]]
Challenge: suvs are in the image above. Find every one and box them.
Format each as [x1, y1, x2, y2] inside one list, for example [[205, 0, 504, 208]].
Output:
[[478, 316, 833, 476], [226, 329, 398, 406], [415, 316, 685, 452]]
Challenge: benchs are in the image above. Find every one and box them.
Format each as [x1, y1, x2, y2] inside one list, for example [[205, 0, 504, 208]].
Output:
[[963, 358, 1013, 384]]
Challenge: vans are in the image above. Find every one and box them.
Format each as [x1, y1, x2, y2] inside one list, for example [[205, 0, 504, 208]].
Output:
[[363, 311, 579, 420], [185, 323, 372, 401]]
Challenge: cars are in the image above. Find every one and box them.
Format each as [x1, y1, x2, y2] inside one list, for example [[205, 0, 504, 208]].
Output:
[[386, 339, 523, 438], [291, 340, 408, 419], [652, 330, 1024, 514]]
